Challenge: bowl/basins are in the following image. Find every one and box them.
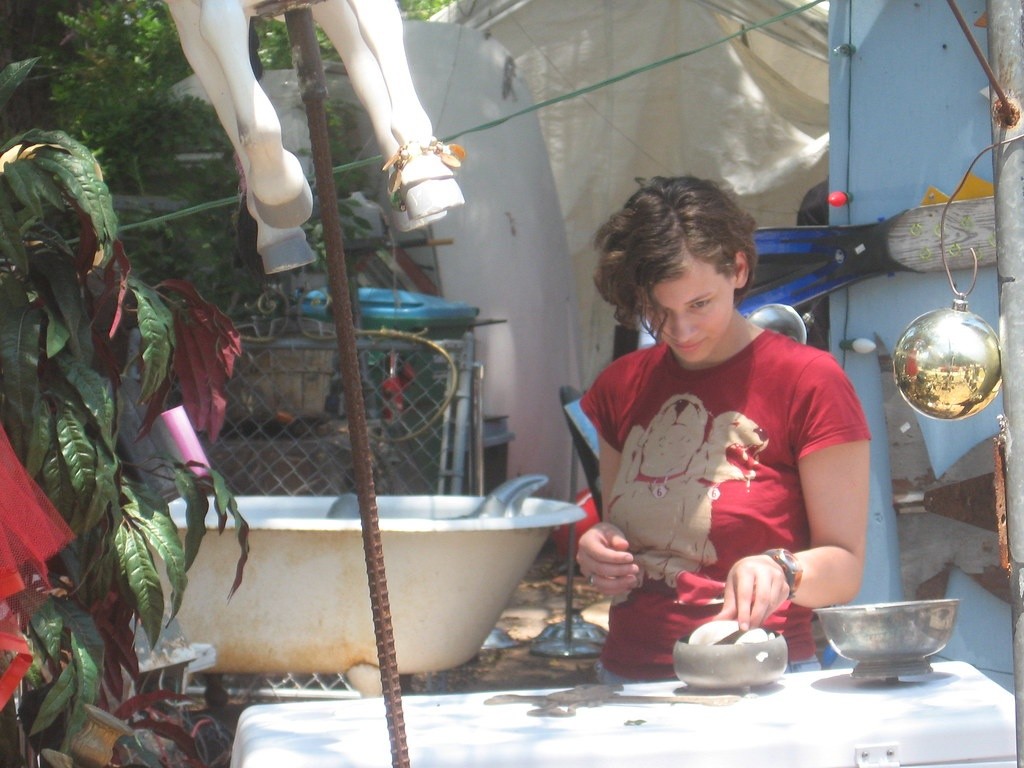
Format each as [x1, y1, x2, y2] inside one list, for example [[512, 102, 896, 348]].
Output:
[[671, 629, 790, 692], [811, 596, 962, 681]]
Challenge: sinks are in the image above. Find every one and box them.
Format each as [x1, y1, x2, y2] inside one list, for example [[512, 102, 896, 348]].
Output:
[[85, 494, 587, 673]]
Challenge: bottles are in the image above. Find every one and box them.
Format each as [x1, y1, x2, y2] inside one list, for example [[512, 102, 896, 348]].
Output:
[[379, 350, 404, 425]]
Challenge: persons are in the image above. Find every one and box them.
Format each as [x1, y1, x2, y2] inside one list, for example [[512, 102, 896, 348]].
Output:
[[577, 176, 871, 685]]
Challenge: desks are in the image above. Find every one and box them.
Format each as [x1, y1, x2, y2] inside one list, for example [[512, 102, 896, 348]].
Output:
[[228, 661, 1018, 768]]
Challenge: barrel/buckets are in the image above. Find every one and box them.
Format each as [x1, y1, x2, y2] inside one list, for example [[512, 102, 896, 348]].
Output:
[[440, 409, 517, 500], [220, 334, 338, 420]]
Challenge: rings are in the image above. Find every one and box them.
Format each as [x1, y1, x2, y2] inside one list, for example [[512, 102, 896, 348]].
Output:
[[587, 575, 594, 586]]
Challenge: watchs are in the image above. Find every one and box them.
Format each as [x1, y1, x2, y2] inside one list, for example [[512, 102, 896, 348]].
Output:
[[765, 548, 804, 600]]
